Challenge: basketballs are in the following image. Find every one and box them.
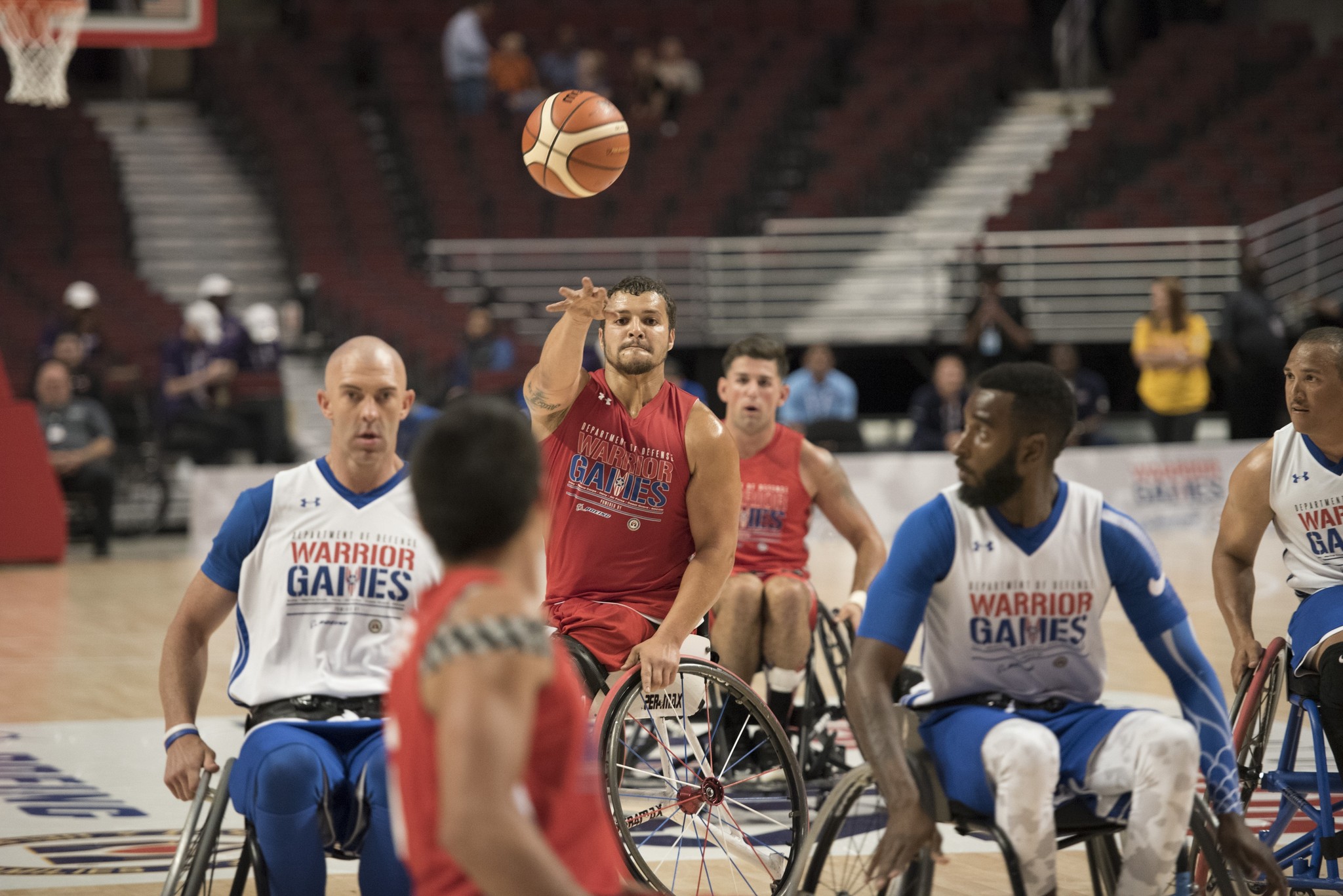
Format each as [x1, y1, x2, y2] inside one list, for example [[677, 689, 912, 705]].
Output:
[[522, 90, 630, 199]]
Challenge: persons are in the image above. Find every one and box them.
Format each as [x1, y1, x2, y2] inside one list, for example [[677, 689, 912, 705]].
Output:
[[29, 258, 1295, 563], [846, 361, 1287, 896], [1130, 276, 1212, 441], [720, 333, 888, 784], [776, 340, 864, 453], [522, 274, 742, 700], [1213, 325, 1343, 781], [382, 395, 668, 896], [157, 334, 445, 896]]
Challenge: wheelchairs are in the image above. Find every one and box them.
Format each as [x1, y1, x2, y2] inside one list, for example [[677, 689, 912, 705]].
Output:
[[688, 584, 887, 809], [542, 626, 811, 896], [783, 690, 1243, 896], [1182, 634, 1343, 896], [158, 694, 415, 896]]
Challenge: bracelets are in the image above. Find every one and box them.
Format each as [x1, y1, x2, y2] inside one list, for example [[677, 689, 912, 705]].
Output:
[[847, 590, 868, 613], [163, 723, 199, 749]]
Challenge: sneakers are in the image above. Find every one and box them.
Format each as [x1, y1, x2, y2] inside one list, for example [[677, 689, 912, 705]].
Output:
[[751, 731, 788, 782], [710, 742, 757, 783]]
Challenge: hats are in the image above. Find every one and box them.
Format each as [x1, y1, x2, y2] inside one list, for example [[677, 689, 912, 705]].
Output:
[[243, 304, 280, 343], [197, 272, 236, 298], [184, 300, 225, 344], [61, 280, 99, 309]]
